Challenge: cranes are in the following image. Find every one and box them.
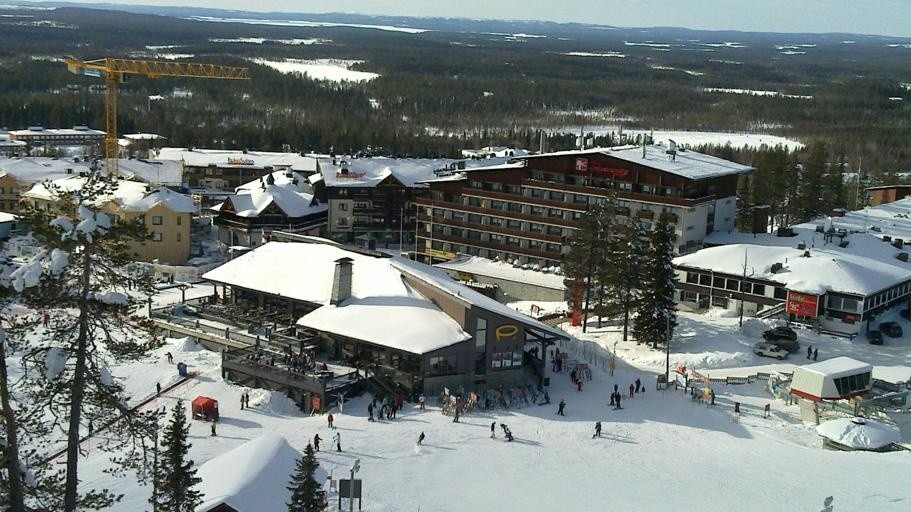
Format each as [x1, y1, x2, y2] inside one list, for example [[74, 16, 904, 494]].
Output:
[[66, 57, 252, 181]]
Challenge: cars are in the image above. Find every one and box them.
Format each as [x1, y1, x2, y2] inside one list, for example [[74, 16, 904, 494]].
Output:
[[753, 326, 799, 360], [900, 309, 911, 321], [868, 330, 883, 344], [879, 322, 903, 338]]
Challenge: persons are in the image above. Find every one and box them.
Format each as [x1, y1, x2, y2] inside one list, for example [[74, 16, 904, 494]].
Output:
[[313, 412, 342, 451], [489, 420, 513, 442], [89, 422, 93, 433], [366, 392, 426, 421], [157, 382, 161, 396], [709, 389, 715, 404], [594, 420, 603, 437], [211, 419, 216, 436], [555, 398, 566, 416], [813, 349, 817, 361], [550, 347, 583, 390], [241, 392, 250, 410], [608, 376, 641, 409], [437, 381, 551, 425], [243, 349, 320, 382], [807, 345, 812, 359], [165, 351, 174, 364]]
